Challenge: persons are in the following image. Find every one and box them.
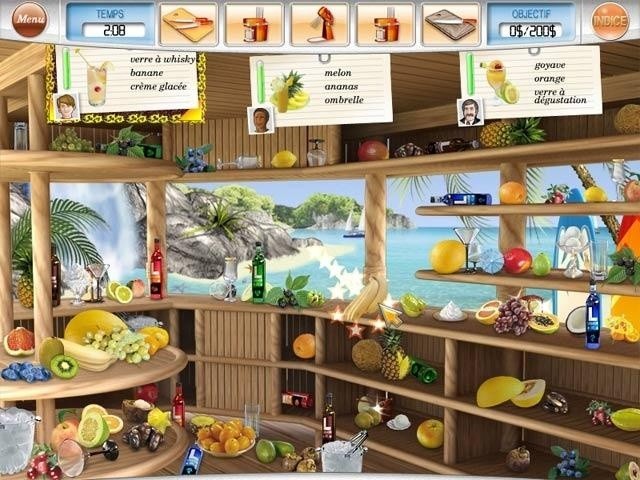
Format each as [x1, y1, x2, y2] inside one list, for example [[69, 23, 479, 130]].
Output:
[[253, 108, 270, 133], [56, 94, 76, 119], [459, 99, 481, 126]]
